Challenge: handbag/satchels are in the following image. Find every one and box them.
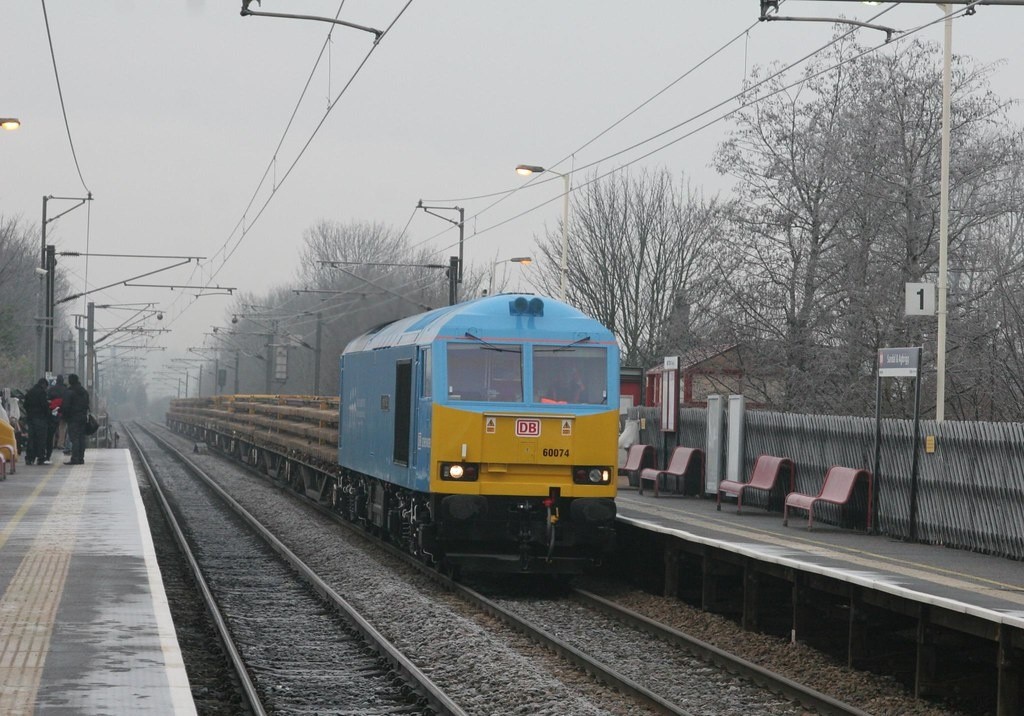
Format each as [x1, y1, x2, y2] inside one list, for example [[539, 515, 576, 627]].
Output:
[[86, 407, 99, 435]]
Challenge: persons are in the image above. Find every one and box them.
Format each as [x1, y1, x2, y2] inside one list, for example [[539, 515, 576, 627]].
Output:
[[538, 365, 585, 404], [0, 387, 23, 454], [22, 372, 90, 466]]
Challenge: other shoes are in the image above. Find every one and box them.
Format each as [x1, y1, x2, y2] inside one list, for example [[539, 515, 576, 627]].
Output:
[[63, 459, 85, 466], [25, 460, 34, 465], [62, 448, 70, 454], [38, 458, 52, 465]]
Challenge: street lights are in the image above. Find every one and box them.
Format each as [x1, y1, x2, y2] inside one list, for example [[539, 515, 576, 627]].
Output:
[[516, 162, 570, 304], [491, 256, 533, 295]]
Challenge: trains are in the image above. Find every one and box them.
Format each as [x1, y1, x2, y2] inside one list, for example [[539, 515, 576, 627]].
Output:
[[165, 288, 622, 598]]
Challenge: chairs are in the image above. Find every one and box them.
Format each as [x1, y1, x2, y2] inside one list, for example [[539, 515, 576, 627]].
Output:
[[717, 454, 795, 515], [618, 445, 655, 488], [784, 465, 874, 531], [638, 445, 705, 498]]
